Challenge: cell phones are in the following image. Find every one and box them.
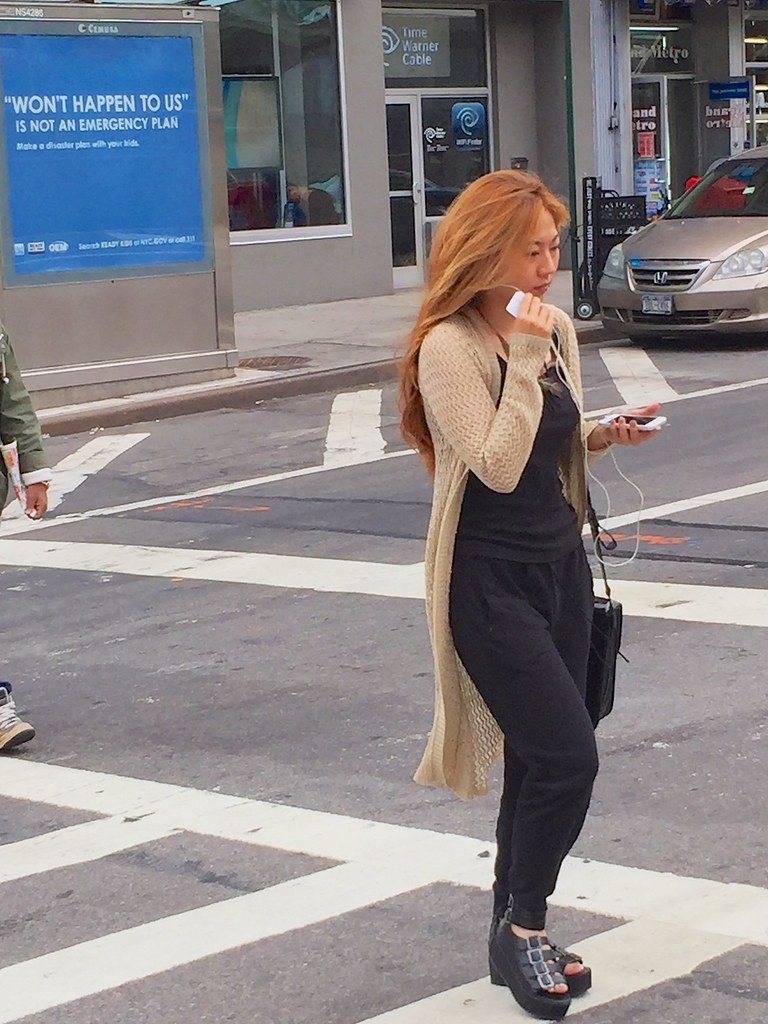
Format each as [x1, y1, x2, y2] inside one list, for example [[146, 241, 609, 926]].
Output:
[[599, 413, 667, 430]]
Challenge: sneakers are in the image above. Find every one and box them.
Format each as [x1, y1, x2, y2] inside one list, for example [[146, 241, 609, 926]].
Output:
[[0, 687, 36, 754]]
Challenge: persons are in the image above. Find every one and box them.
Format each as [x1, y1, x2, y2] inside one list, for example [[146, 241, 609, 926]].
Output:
[[398, 172, 661, 1019], [0, 319, 54, 750], [283, 177, 338, 225]]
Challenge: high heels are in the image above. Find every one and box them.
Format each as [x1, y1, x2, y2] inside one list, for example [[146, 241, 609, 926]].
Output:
[[484, 915, 572, 1022], [549, 941, 592, 996]]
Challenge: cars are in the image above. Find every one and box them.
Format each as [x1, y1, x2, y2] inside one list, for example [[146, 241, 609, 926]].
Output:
[[597, 140, 768, 341]]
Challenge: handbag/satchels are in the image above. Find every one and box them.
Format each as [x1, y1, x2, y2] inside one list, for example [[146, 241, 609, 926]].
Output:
[[583, 595, 624, 727]]
